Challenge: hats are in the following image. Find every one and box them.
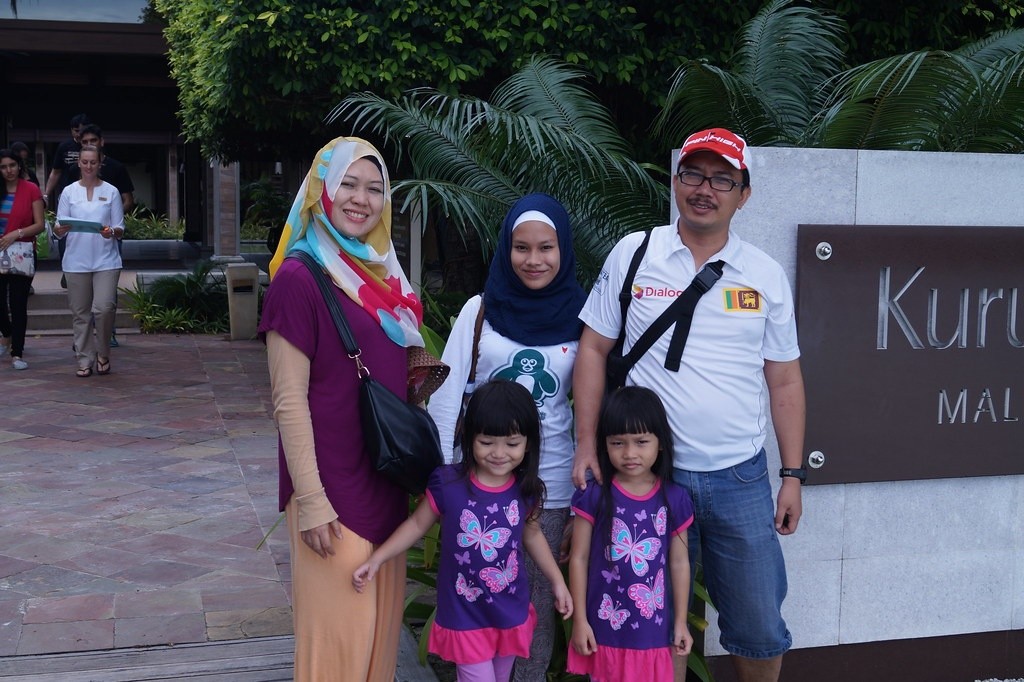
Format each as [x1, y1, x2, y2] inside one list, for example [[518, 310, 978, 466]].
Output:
[[678, 128, 752, 185]]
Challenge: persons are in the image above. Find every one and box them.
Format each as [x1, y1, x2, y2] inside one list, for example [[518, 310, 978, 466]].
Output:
[[9, 142, 40, 294], [261, 138, 426, 682], [353, 380, 570, 682], [0, 150, 45, 370], [47, 115, 133, 377], [572, 128, 805, 682], [430, 193, 592, 682], [572, 386, 693, 682]]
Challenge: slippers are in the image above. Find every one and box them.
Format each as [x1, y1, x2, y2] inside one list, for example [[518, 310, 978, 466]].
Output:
[[96, 352, 110, 374], [76, 367, 93, 377]]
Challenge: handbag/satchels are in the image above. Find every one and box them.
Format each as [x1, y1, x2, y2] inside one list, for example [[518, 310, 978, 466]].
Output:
[[359, 374, 445, 497], [452, 293, 484, 446], [0, 242, 35, 277]]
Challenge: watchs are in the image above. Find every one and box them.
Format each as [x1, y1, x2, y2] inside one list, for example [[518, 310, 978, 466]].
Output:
[[18, 229, 24, 238], [779, 466, 805, 486], [111, 228, 116, 237]]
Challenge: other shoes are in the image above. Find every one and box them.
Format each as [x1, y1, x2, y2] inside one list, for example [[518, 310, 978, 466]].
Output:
[[12, 358, 28, 369], [0, 343, 9, 356], [29, 286, 34, 294], [109, 333, 119, 346], [61, 274, 67, 288]]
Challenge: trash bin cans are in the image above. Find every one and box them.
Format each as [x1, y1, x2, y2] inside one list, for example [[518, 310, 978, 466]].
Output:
[[225, 263, 259, 340]]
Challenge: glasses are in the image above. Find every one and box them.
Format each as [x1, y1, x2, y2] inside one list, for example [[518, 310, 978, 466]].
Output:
[[678, 171, 744, 192]]
